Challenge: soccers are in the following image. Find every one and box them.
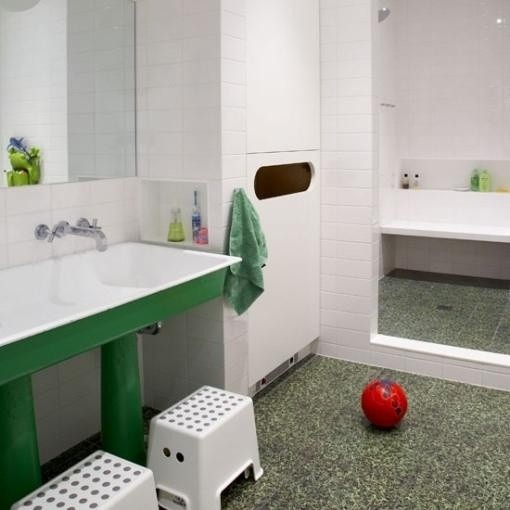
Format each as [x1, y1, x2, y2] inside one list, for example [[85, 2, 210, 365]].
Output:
[[362, 379, 411, 429]]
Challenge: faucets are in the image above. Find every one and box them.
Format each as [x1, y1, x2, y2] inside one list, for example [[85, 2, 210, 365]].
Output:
[[51, 219, 107, 255]]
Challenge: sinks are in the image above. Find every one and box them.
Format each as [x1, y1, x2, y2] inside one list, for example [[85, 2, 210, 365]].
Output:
[[0, 258, 146, 389], [98, 240, 243, 324]]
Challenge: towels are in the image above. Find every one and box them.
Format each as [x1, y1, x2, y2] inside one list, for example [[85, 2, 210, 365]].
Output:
[[226, 186, 272, 316]]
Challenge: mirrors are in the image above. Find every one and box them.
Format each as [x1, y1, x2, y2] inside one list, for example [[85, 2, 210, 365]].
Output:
[[0, 0, 138, 188]]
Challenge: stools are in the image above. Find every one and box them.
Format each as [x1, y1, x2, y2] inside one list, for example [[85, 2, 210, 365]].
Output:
[[13, 385, 266, 509]]
